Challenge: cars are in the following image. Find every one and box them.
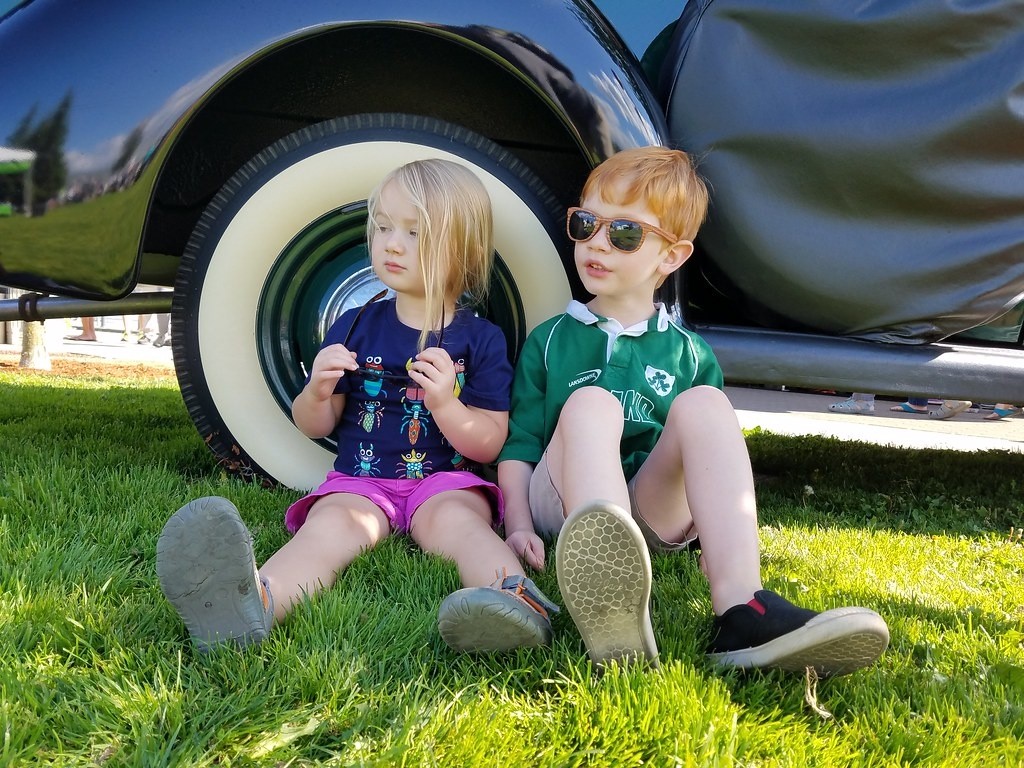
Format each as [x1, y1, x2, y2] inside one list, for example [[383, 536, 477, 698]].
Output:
[[0, 0, 1024, 506]]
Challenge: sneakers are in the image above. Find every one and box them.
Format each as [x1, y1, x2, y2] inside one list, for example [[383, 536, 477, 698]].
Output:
[[156, 497, 277, 656], [434, 576, 560, 654], [828, 399, 876, 415], [704, 588, 889, 678], [927, 401, 972, 420], [556, 502, 659, 669]]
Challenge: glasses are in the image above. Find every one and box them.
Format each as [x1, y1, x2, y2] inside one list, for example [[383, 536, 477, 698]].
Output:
[[339, 289, 446, 387], [568, 204, 678, 254]]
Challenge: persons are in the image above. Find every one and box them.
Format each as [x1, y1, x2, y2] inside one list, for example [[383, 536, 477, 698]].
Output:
[[156, 159, 561, 659], [494, 146, 890, 680], [69, 317, 97, 341], [121, 314, 171, 347]]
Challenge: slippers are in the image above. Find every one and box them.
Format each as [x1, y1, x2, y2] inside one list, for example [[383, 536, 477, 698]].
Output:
[[891, 402, 931, 415], [984, 404, 1015, 421]]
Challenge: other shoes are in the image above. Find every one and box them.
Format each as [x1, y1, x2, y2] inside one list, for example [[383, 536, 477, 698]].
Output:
[[70, 333, 97, 342], [137, 335, 151, 344], [153, 335, 173, 347], [121, 332, 131, 341]]
